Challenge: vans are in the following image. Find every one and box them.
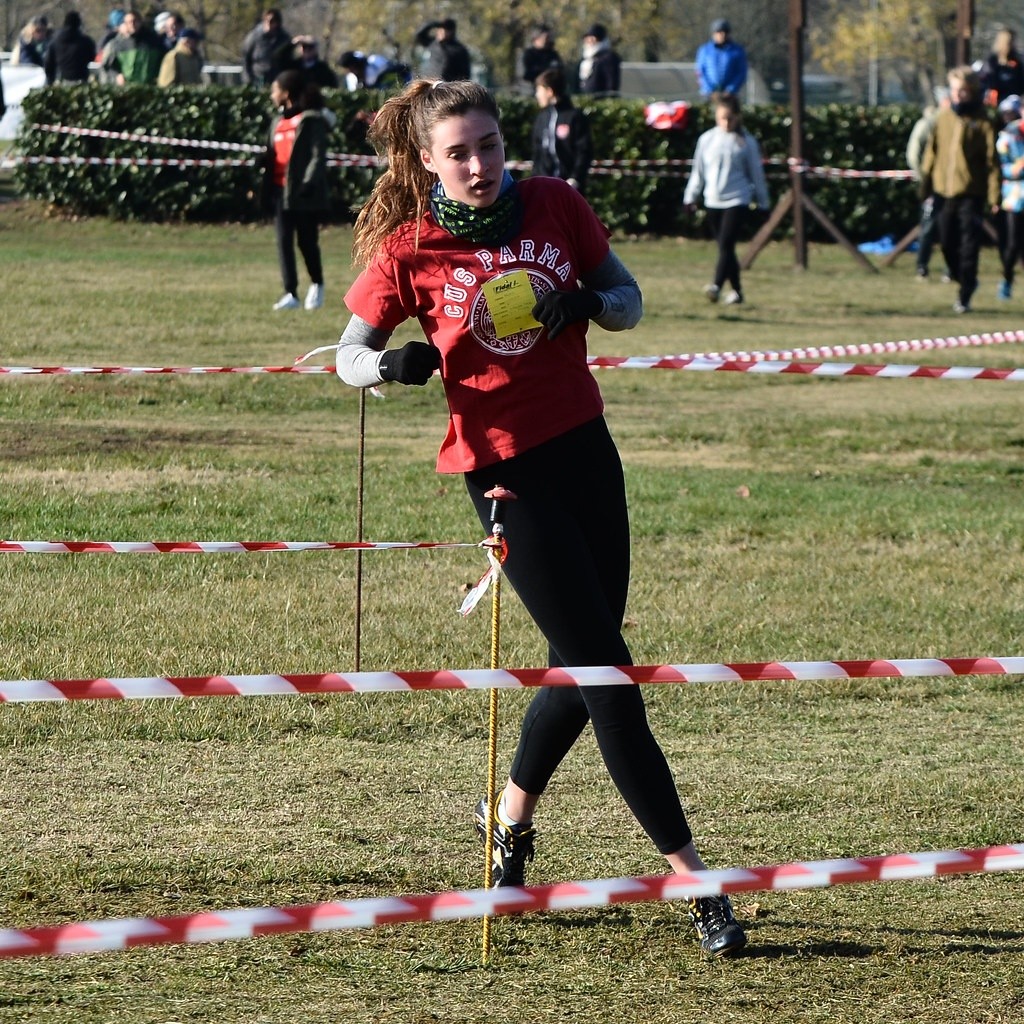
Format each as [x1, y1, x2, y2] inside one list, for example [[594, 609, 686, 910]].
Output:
[[617, 58, 772, 110]]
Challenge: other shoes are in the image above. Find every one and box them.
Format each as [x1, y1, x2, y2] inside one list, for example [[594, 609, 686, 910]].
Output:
[[705, 285, 718, 303], [918, 267, 929, 281], [945, 270, 954, 280], [998, 280, 1012, 299], [955, 301, 971, 313], [304, 283, 323, 311], [472, 788, 537, 889], [726, 291, 742, 305], [272, 292, 299, 310]]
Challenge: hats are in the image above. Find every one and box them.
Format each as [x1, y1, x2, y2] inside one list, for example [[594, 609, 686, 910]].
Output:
[[582, 24, 607, 44], [154, 11, 171, 31], [997, 94, 1022, 113], [709, 17, 729, 36], [108, 9, 126, 30], [177, 28, 202, 41]]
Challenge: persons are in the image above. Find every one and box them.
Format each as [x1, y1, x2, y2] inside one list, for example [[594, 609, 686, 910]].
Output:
[[8, 15, 51, 68], [417, 19, 470, 81], [100, 10, 202, 87], [266, 72, 328, 309], [682, 97, 769, 309], [696, 18, 748, 101], [243, 8, 295, 87], [575, 24, 621, 95], [337, 79, 747, 955], [44, 12, 95, 84], [523, 25, 563, 80], [277, 32, 338, 86], [906, 30, 1024, 314], [336, 51, 410, 88], [528, 70, 592, 194]]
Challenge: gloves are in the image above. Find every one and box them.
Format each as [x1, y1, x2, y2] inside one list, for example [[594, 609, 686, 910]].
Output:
[[532, 290, 603, 339], [378, 341, 440, 386]]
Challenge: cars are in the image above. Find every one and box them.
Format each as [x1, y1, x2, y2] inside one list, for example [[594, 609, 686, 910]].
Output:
[[0, 50, 125, 145]]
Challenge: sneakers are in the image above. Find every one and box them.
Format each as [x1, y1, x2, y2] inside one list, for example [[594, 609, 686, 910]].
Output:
[[686, 892, 747, 958]]
